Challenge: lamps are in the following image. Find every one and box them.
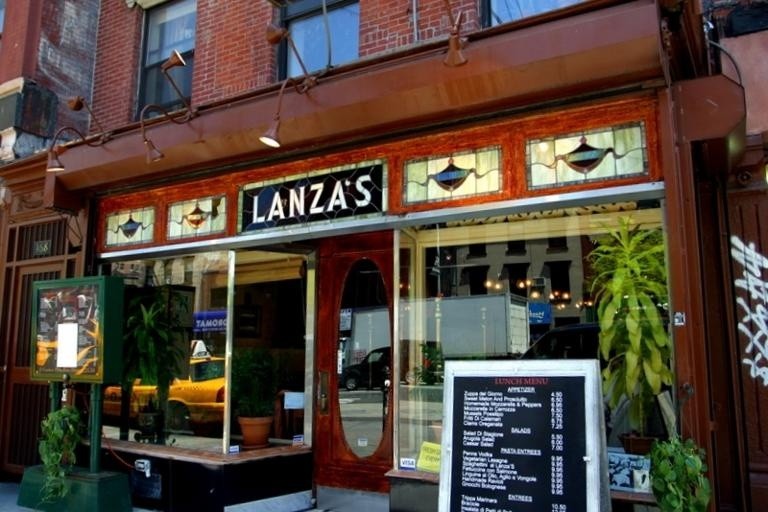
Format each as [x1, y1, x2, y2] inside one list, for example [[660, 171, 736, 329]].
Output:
[[439, 0, 473, 69], [135, 44, 201, 168], [43, 90, 112, 172], [256, 17, 334, 152]]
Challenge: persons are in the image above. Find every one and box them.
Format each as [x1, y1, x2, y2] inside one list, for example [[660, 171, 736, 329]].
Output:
[[639, 474, 649, 491]]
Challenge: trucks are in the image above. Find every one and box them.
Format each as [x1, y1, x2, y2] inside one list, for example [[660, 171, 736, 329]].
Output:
[[337, 291, 532, 389]]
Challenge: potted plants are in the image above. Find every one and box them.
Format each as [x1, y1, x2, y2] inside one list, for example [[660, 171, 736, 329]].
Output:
[[581, 209, 677, 458], [229, 343, 288, 452]]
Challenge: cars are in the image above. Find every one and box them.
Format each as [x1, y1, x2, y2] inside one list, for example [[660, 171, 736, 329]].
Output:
[[102, 355, 226, 431], [340, 346, 392, 392]]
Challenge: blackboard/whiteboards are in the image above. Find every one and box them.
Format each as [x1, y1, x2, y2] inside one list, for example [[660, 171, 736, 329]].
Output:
[[438, 359, 599, 511]]
